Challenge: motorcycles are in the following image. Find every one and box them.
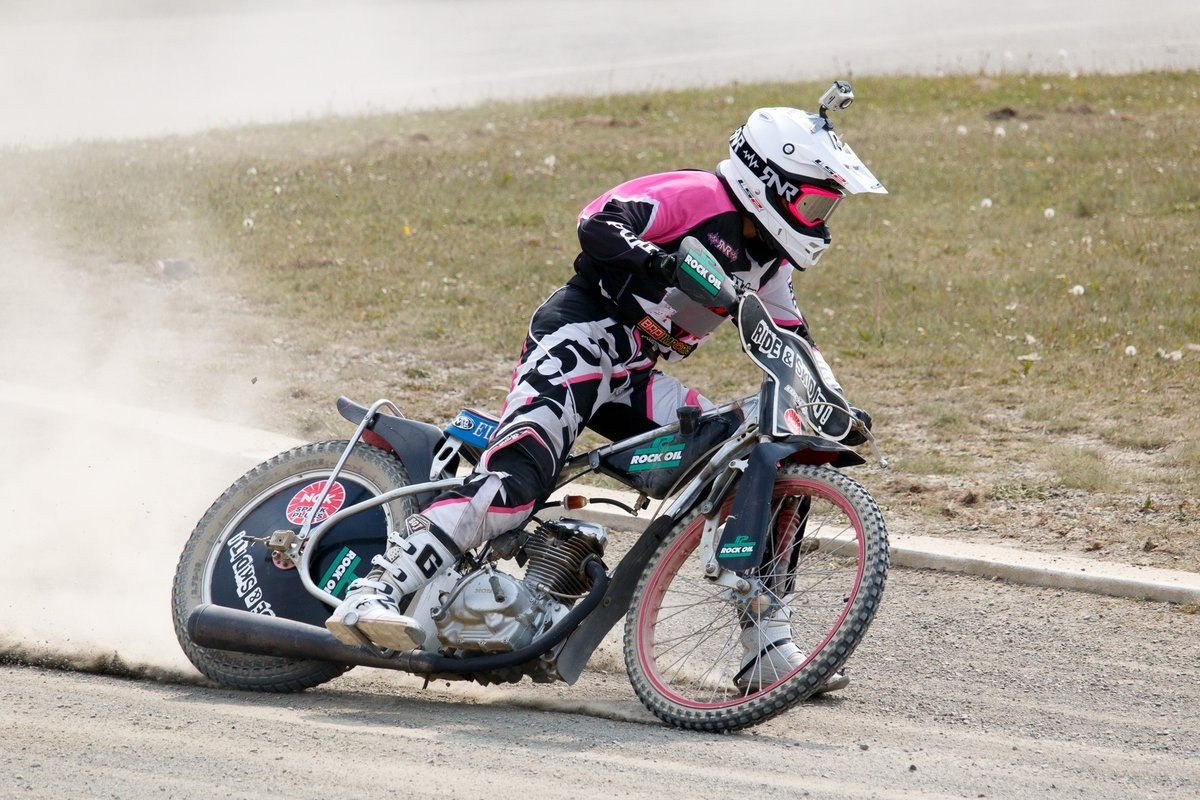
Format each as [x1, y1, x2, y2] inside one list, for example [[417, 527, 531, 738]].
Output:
[[169, 235, 893, 736]]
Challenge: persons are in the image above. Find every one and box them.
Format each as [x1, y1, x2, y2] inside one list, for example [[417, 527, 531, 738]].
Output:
[[327, 107, 890, 695]]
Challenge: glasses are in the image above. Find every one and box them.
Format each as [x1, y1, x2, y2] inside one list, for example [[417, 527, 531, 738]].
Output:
[[787, 182, 847, 229]]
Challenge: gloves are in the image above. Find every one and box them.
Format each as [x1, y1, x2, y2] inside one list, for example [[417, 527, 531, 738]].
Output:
[[844, 407, 874, 446], [653, 251, 678, 285]]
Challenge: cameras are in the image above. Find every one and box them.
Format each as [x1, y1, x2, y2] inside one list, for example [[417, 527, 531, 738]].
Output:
[[818, 80, 855, 111]]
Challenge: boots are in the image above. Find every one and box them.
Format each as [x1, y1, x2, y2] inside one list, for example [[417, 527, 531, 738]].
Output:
[[730, 594, 851, 694], [325, 513, 465, 646]]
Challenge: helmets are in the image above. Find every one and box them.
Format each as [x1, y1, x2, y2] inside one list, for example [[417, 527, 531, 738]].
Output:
[[720, 107, 889, 274]]
